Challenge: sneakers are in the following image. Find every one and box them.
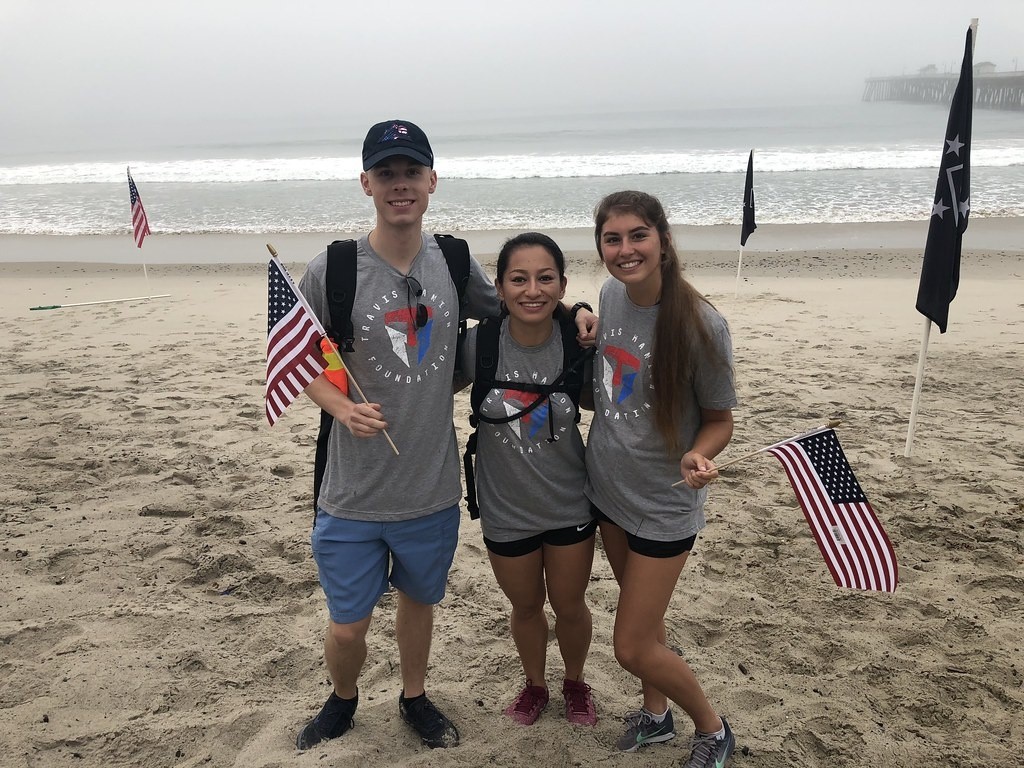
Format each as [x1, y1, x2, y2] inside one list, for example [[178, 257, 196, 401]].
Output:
[[682, 714, 736, 768], [561, 678, 597, 727], [613, 707, 676, 753], [398, 688, 460, 750], [297, 686, 359, 750], [504, 679, 549, 726]]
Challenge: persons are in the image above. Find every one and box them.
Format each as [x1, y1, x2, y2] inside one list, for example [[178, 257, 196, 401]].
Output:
[[297, 120, 600, 751], [579, 191, 735, 768]]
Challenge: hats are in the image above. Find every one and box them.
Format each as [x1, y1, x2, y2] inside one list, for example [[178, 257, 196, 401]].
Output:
[[361, 119, 432, 171]]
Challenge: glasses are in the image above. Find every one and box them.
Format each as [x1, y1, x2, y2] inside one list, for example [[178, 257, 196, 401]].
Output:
[[402, 275, 430, 332]]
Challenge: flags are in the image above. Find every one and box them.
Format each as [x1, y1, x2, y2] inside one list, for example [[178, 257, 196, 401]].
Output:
[[763, 420, 898, 594], [915, 18, 980, 334], [740, 148, 758, 246], [128, 175, 151, 248], [264, 257, 329, 427]]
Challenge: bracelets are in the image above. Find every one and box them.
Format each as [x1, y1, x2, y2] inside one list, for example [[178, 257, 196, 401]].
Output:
[[570, 302, 593, 323]]
